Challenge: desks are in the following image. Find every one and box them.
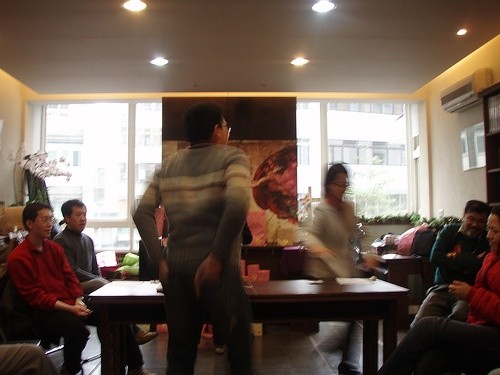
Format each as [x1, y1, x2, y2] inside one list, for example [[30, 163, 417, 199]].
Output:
[[88, 277, 411, 375]]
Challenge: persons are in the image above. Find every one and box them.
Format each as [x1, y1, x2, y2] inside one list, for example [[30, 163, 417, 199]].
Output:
[[299, 162, 388, 375], [376, 203, 500, 375], [6, 202, 120, 375], [131, 100, 255, 375], [406, 199, 490, 328], [196, 213, 253, 354], [54, 198, 160, 375]]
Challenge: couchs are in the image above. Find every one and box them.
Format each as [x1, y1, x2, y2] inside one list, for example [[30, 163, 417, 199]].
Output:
[[360, 223, 442, 327]]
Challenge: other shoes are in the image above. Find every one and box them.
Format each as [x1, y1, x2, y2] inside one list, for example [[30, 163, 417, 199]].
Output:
[[128, 366, 151, 375], [214, 347, 225, 354], [337, 362, 361, 375], [135, 328, 158, 346]]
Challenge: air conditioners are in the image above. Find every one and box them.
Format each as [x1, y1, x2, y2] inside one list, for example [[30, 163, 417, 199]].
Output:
[[439, 68, 495, 114]]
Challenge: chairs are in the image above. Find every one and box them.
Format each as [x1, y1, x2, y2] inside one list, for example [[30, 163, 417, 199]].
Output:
[[0, 270, 102, 375]]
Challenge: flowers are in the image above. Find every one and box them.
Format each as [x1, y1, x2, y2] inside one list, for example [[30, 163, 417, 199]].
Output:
[[7, 143, 72, 202]]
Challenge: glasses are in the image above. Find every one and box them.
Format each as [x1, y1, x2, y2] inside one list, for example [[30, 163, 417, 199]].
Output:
[[331, 182, 349, 188]]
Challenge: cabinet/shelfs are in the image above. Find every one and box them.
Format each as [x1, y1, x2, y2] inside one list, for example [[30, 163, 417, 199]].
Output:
[[474, 80, 500, 208]]
